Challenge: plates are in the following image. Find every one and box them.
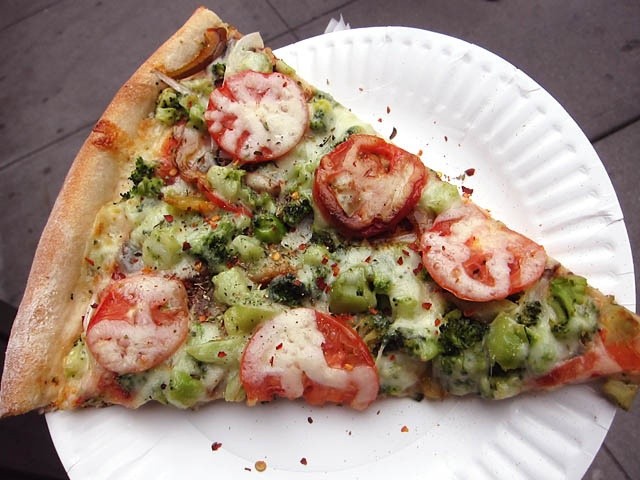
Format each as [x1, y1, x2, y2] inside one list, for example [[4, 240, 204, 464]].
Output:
[[44, 23, 637, 480]]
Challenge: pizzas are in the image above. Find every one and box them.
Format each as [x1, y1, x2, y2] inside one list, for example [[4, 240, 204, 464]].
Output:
[[0, 5, 640, 421]]
